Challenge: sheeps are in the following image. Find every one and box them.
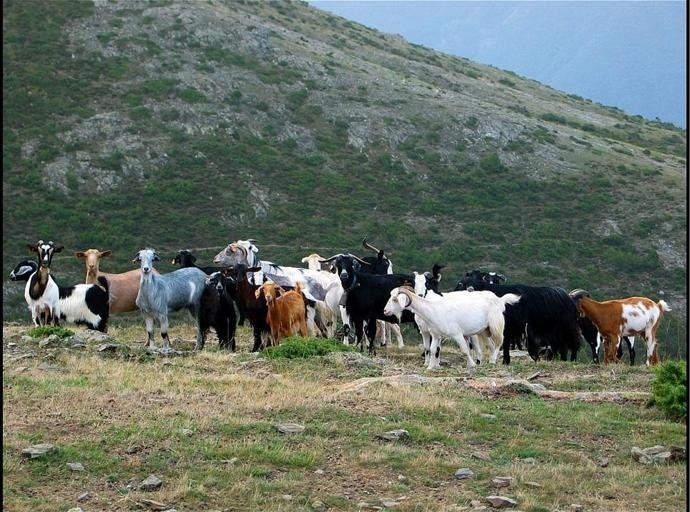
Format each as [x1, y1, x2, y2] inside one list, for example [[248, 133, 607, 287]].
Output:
[[9, 233, 674, 373]]
